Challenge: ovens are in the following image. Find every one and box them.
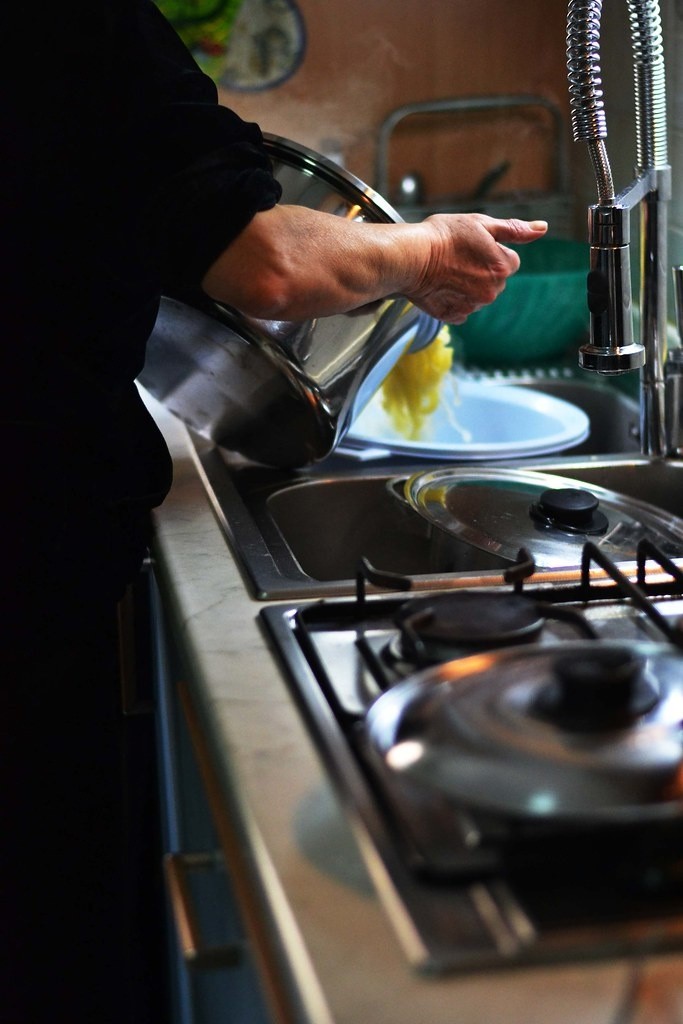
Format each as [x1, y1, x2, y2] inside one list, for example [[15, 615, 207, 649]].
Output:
[[153, 701, 289, 1022]]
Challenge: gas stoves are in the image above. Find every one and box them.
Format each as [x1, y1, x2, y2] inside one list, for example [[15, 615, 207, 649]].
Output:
[[259, 537, 683, 973]]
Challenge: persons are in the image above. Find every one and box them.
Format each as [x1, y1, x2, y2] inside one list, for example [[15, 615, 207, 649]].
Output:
[[0, 0, 549, 1024]]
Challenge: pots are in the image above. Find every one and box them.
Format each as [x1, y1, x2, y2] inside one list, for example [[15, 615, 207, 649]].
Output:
[[334, 381, 591, 463], [374, 463, 682, 574], [138, 124, 446, 474]]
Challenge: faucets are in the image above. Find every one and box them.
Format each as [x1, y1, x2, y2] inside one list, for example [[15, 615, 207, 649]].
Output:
[[566, 0, 683, 456]]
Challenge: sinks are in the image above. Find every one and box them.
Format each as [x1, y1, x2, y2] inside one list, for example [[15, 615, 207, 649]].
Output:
[[232, 375, 642, 468], [265, 463, 683, 584]]
